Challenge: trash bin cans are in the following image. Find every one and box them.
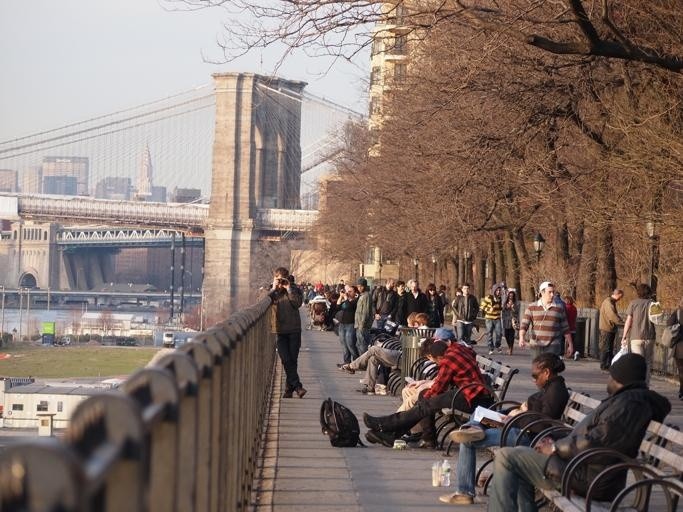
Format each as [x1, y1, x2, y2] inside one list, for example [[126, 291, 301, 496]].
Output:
[[399, 328, 437, 400], [573, 317, 587, 357]]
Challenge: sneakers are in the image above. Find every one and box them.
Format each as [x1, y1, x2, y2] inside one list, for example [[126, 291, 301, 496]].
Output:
[[282, 389, 293, 399], [573, 351, 580, 361], [446, 427, 488, 445], [489, 350, 495, 356], [354, 386, 374, 396], [364, 429, 395, 449], [296, 386, 307, 399], [409, 438, 437, 450], [374, 384, 389, 396], [336, 361, 356, 376], [437, 490, 475, 506], [495, 347, 503, 354], [362, 411, 384, 432]]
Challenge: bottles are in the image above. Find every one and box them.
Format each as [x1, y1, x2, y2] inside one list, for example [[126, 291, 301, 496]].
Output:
[[431, 462, 440, 487], [440, 458, 451, 487]]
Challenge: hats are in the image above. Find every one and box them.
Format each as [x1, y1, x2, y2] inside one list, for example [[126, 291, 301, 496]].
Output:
[[608, 351, 648, 387], [429, 326, 456, 340], [538, 281, 554, 299], [356, 278, 369, 289]]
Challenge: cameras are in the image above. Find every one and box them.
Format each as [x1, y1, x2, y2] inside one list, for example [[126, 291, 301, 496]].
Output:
[[278, 277, 289, 286]]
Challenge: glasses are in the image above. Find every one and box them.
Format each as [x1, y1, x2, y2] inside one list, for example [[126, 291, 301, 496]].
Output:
[[530, 370, 543, 381]]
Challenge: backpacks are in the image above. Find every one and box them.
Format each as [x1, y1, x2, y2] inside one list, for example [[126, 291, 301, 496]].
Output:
[[319, 396, 360, 448]]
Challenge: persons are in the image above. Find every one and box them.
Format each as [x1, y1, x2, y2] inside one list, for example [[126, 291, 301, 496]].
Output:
[[266, 265, 682, 512]]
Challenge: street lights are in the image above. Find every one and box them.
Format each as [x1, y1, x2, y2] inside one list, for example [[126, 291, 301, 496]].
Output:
[[530, 231, 545, 300], [644, 220, 662, 306], [414, 256, 418, 294], [460, 249, 473, 298]]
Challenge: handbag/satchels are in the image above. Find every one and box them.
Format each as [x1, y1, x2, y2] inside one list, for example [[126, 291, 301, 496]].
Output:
[[511, 313, 521, 332], [661, 322, 682, 349], [332, 310, 345, 325]]
